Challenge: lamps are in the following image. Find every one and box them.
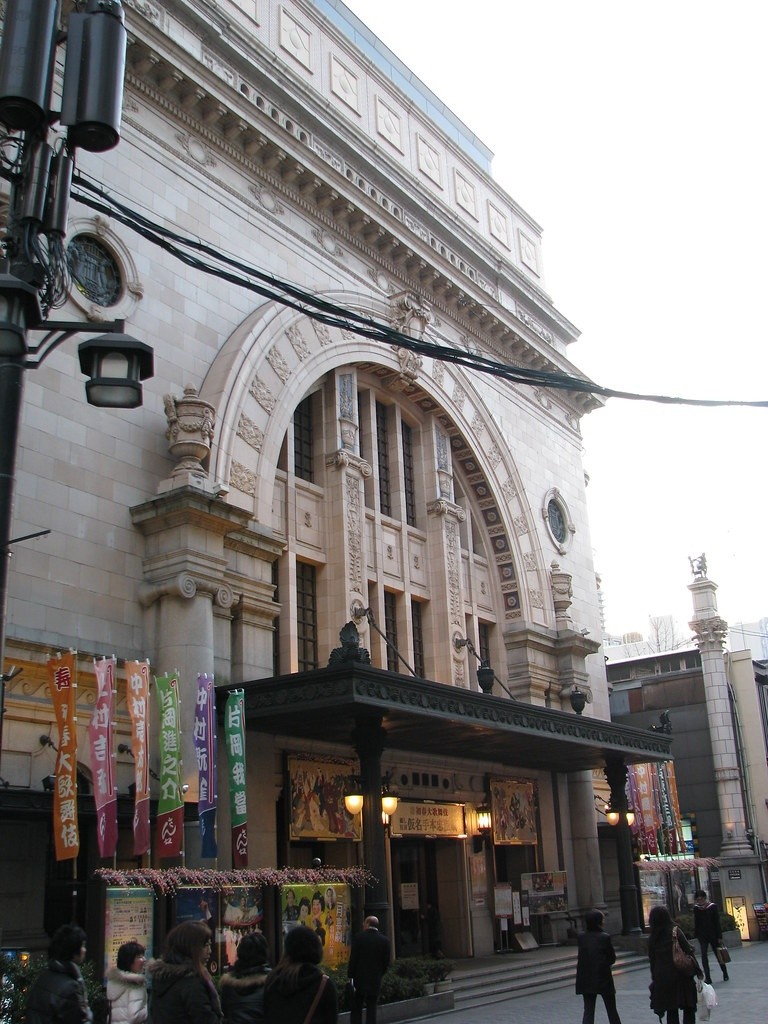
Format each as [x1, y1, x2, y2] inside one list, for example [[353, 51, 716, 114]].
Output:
[[726, 831, 732, 838], [472, 799, 492, 853], [342, 772, 365, 817], [626, 808, 636, 826], [382, 779, 399, 830], [606, 793, 620, 827]]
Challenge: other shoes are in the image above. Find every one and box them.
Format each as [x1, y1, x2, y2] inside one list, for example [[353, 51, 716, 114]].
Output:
[[724, 975, 729, 981], [704, 979, 712, 984]]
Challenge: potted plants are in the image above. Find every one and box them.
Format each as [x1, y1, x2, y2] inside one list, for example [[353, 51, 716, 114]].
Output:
[[407, 956, 460, 995]]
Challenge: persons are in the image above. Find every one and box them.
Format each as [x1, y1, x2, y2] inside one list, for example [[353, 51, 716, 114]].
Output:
[[675, 882, 687, 911], [200, 888, 336, 972], [689, 557, 698, 573], [648, 907, 705, 1024], [27, 922, 94, 1024], [693, 891, 730, 983], [698, 552, 708, 579], [219, 932, 275, 1024], [262, 926, 338, 1024], [575, 909, 624, 1024], [105, 941, 150, 1024], [349, 915, 391, 1024], [148, 920, 224, 1024]]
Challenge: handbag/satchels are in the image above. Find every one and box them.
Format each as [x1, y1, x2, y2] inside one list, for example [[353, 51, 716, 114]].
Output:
[[648, 964, 669, 1023], [717, 943, 731, 964], [672, 926, 698, 974], [697, 980, 717, 1021], [345, 978, 360, 1010]]
